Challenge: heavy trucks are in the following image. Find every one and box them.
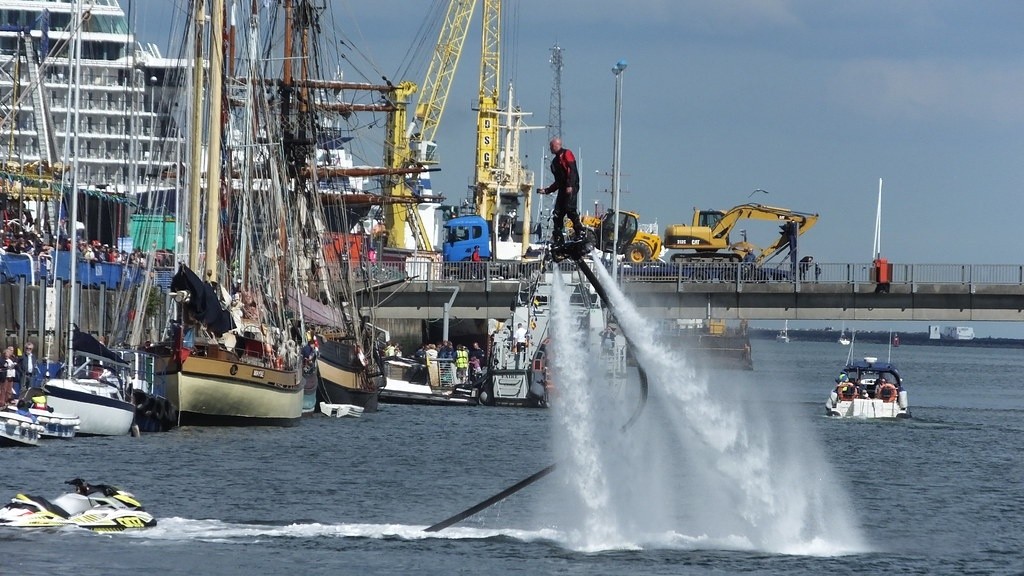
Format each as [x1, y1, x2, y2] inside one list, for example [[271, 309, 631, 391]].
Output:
[[443, 214, 791, 285]]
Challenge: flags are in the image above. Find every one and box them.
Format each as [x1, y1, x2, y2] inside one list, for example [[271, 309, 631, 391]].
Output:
[[534, 299, 539, 309], [531, 319, 536, 330]]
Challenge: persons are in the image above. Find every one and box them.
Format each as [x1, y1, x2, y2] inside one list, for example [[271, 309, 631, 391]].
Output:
[[814, 263, 822, 284], [385, 342, 403, 358], [472, 245, 485, 280], [302, 340, 317, 374], [0, 346, 16, 411], [875, 379, 886, 399], [835, 372, 849, 396], [536, 138, 586, 248], [514, 323, 527, 362], [799, 255, 814, 284], [168, 320, 181, 346], [15, 400, 39, 425], [15, 342, 38, 397], [7, 346, 25, 400], [416, 341, 484, 372], [0, 227, 149, 268]]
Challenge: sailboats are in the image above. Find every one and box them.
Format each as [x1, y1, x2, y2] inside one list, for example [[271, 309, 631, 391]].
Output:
[[0, 0, 393, 446]]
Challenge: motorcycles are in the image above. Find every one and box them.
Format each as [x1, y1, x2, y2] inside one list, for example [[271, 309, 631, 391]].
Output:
[[0, 477, 160, 533]]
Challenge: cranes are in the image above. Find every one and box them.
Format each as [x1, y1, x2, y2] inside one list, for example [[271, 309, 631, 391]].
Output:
[[388, 0, 523, 256]]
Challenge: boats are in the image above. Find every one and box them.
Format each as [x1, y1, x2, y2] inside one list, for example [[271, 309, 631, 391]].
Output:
[[825, 362, 909, 421]]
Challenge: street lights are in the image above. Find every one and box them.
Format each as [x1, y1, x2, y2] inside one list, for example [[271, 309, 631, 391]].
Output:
[[612, 60, 629, 284]]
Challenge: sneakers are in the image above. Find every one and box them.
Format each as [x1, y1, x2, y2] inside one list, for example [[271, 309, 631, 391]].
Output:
[[573, 223, 587, 243], [550, 230, 565, 249]]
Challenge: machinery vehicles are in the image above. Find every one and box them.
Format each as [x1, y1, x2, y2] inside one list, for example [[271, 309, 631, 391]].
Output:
[[563, 200, 819, 267]]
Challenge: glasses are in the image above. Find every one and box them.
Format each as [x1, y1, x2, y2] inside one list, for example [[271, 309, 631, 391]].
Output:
[[26, 348, 32, 350], [881, 381, 886, 383]]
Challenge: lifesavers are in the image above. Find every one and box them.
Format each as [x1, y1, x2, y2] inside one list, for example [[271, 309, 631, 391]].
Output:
[[878, 384, 897, 404], [839, 382, 857, 399]]
